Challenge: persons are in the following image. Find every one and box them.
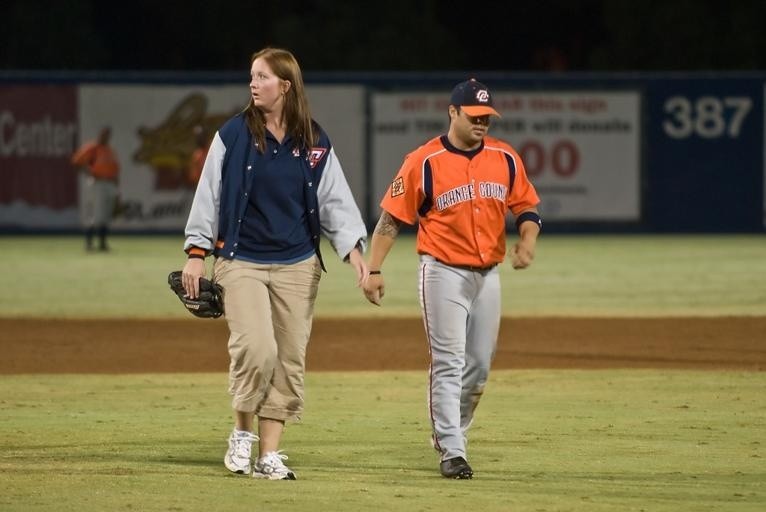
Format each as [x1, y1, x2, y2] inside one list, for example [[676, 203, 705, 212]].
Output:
[[183, 47, 371, 480], [70, 125, 130, 251], [365, 77, 541, 477]]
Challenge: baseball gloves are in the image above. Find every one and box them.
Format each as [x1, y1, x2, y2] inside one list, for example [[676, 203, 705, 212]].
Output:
[[167, 269, 226, 318]]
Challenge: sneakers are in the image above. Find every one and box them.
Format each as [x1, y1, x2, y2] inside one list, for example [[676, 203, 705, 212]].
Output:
[[223, 425, 260, 476], [252, 449, 296, 481], [439, 457, 474, 480]]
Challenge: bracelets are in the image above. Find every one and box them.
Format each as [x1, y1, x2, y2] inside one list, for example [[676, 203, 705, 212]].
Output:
[[369, 270, 381, 274]]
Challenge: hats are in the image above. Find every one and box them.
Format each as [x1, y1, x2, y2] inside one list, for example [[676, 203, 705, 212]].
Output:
[[450, 79, 502, 120]]
